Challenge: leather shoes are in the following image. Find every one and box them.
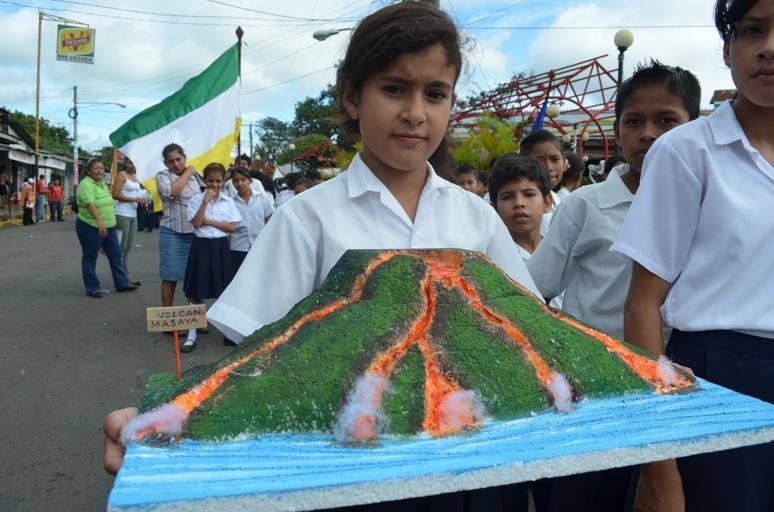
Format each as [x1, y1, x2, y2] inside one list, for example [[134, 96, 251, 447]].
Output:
[[180, 339, 196, 352], [117, 280, 141, 292], [92, 290, 103, 297], [224, 337, 236, 346]]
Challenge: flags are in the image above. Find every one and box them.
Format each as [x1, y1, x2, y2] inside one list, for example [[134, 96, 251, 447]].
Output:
[[109, 44, 240, 214]]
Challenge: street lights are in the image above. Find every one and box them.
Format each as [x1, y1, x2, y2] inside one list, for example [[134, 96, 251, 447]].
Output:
[[254, 154, 261, 169], [563, 123, 589, 153], [288, 143, 296, 173], [608, 27, 636, 90], [311, 26, 356, 43], [531, 69, 560, 130], [72, 98, 127, 190]]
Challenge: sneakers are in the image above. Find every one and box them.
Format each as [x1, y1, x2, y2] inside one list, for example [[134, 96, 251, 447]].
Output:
[[169, 330, 188, 336], [196, 329, 209, 334]]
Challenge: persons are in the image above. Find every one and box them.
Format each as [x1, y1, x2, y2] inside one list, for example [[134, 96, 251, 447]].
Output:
[[525, 58, 701, 355], [461, 134, 628, 307], [608, 0, 774, 512], [1, 151, 154, 300], [157, 143, 322, 352], [104, 1, 545, 510]]
